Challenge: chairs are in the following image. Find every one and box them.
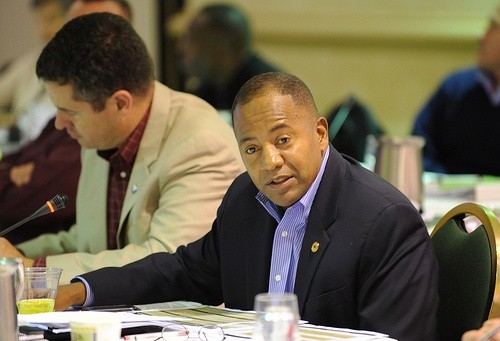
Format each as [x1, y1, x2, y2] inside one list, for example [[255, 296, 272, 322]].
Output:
[[429, 203, 500, 341]]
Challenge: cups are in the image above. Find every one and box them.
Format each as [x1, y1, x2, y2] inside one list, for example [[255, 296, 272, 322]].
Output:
[[69, 313, 122, 340], [16, 268, 62, 314], [375, 135, 426, 214], [253, 293, 301, 341]]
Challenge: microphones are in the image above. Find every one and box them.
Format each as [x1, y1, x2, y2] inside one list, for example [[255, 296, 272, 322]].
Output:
[[0, 192, 70, 237]]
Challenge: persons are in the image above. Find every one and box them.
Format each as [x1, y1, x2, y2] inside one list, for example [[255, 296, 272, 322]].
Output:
[[0, 11, 251, 287], [410, 6, 500, 176], [1, 0, 134, 159], [461, 318, 500, 341], [27, 72, 439, 341], [0, 0, 74, 130], [172, 3, 283, 112]]
